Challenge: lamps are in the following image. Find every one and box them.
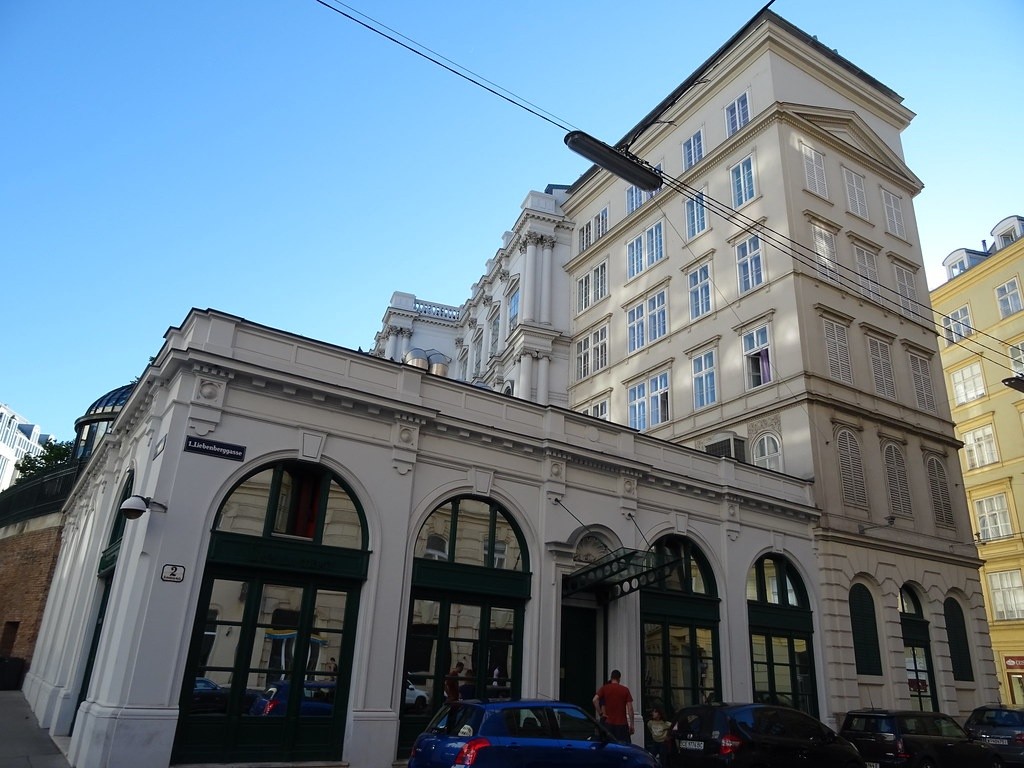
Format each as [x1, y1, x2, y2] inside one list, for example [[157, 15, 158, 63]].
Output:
[[858, 516, 896, 534], [949, 541, 986, 548]]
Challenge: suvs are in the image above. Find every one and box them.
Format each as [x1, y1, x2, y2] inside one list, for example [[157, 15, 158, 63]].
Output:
[[963, 703, 1024, 767]]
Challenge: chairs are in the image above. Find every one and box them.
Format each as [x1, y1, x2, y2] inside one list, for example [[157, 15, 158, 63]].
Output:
[[1004, 714, 1017, 722], [915, 719, 942, 735], [523, 718, 537, 728]]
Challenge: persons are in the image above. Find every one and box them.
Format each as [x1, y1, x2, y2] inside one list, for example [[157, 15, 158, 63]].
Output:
[[446, 662, 476, 700], [647, 705, 671, 761], [492, 666, 508, 685], [593, 670, 635, 743], [328, 657, 337, 681]]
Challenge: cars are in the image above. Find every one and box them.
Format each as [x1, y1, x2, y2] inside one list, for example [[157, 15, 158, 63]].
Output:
[[189, 677, 255, 715], [251, 678, 335, 718], [408, 698, 662, 768], [659, 702, 867, 768], [839, 706, 1007, 768], [405, 677, 430, 714]]
